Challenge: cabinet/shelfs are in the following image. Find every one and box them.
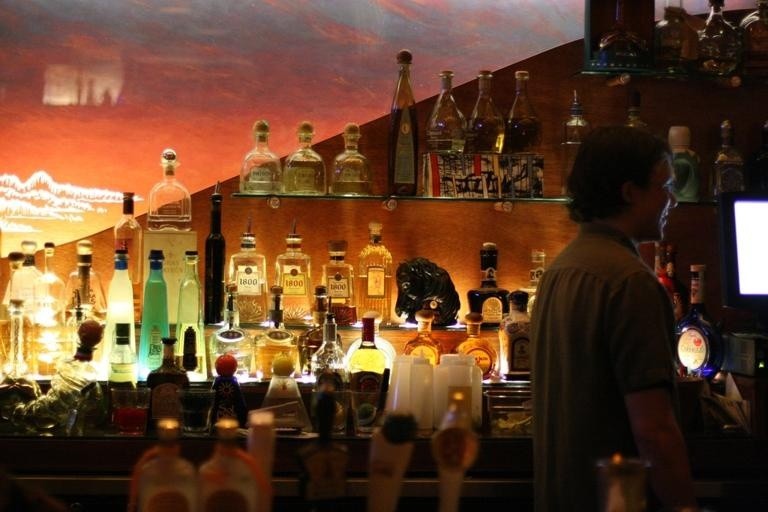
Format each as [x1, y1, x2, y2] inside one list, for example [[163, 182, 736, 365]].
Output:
[[135, 68, 744, 331]]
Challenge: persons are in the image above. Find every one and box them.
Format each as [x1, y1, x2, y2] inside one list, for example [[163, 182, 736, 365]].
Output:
[[670, 276, 728, 384], [529, 125, 700, 511]]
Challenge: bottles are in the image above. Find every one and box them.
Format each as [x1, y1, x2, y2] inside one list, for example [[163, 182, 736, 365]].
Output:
[[0, 0, 768, 510]]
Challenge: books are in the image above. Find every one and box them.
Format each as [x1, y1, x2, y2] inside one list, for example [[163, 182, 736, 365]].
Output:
[[700, 391, 755, 438]]
